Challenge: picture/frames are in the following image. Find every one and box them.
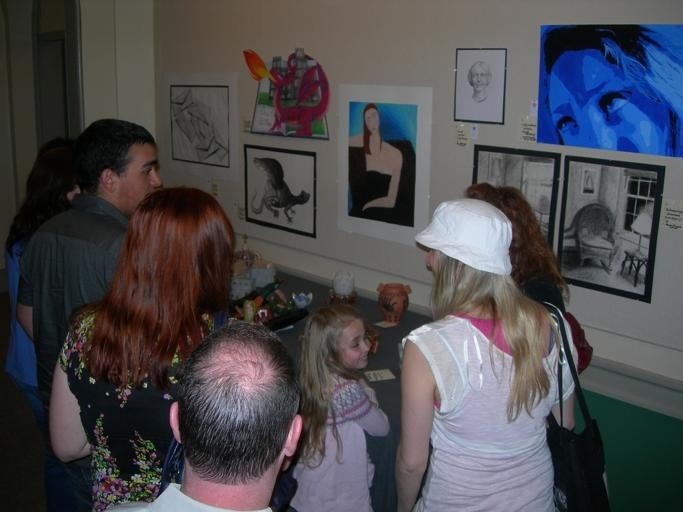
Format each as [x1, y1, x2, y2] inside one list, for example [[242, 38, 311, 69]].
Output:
[[556, 154, 667, 306], [470, 144, 560, 259], [451, 47, 505, 126], [242, 145, 317, 239]]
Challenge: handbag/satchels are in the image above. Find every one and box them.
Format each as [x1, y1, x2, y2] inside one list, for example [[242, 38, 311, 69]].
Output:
[[565, 311, 593, 375], [270, 471, 297, 512], [547, 419, 612, 512]]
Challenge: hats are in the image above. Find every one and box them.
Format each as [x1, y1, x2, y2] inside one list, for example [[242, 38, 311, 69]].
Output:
[[414, 198, 513, 275]]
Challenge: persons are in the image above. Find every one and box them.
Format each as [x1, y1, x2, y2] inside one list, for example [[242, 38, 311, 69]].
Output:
[[3, 138, 81, 512], [467, 62, 492, 107], [49, 187, 238, 512], [348, 102, 404, 222], [464, 183, 570, 319], [102, 320, 303, 512], [14, 120, 161, 512], [394, 198, 578, 512], [281, 303, 390, 512], [541, 25, 683, 158]]
